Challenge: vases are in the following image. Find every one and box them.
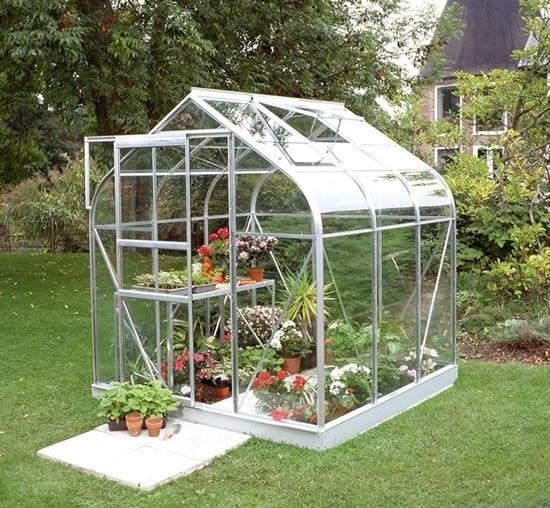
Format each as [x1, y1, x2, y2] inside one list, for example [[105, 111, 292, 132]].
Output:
[[249, 266, 265, 282]]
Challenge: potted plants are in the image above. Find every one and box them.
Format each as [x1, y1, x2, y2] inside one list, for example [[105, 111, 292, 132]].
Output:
[[97, 377, 183, 439], [277, 255, 337, 370], [130, 266, 220, 297]]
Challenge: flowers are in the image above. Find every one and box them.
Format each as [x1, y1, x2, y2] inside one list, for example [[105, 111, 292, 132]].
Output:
[[156, 304, 439, 425], [195, 226, 280, 272]]
